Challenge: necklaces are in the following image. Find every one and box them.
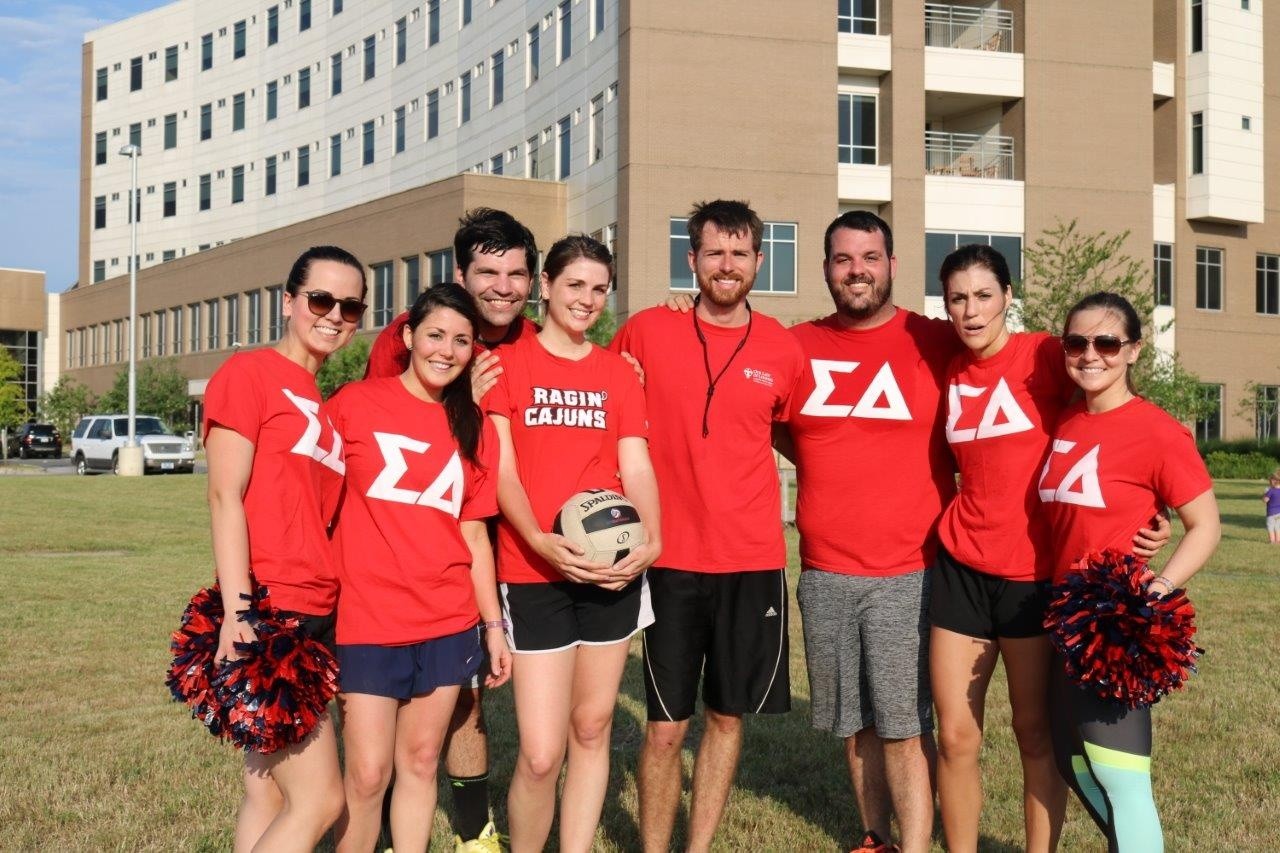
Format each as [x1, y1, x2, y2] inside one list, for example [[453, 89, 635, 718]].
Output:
[[693, 292, 752, 436]]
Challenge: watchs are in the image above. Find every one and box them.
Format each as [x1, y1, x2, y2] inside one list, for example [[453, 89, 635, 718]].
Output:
[[483, 619, 509, 629]]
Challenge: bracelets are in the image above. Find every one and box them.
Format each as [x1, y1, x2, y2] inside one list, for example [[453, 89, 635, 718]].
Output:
[[1153, 576, 1174, 593]]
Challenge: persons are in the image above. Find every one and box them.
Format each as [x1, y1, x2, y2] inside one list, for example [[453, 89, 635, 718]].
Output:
[[1039, 291, 1220, 853], [471, 198, 795, 853], [1263, 469, 1280, 543], [653, 210, 970, 853], [925, 248, 1169, 853], [367, 211, 645, 853], [325, 285, 514, 853], [487, 236, 658, 853], [205, 246, 366, 853]]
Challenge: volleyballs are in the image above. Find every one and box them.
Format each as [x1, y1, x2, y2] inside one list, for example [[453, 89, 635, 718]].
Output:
[[551, 487, 650, 570]]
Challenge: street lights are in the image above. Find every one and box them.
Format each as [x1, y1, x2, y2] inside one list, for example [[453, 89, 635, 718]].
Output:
[[120, 145, 143, 476]]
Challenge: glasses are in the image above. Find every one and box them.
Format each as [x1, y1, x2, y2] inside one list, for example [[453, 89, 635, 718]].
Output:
[[1061, 335, 1130, 357], [295, 289, 366, 324]]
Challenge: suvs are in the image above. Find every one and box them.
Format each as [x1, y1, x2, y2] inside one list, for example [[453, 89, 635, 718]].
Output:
[[7, 423, 63, 458], [71, 415, 195, 477]]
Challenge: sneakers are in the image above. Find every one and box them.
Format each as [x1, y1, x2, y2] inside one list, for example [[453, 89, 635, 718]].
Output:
[[849, 830, 901, 853], [453, 821, 511, 853]]
